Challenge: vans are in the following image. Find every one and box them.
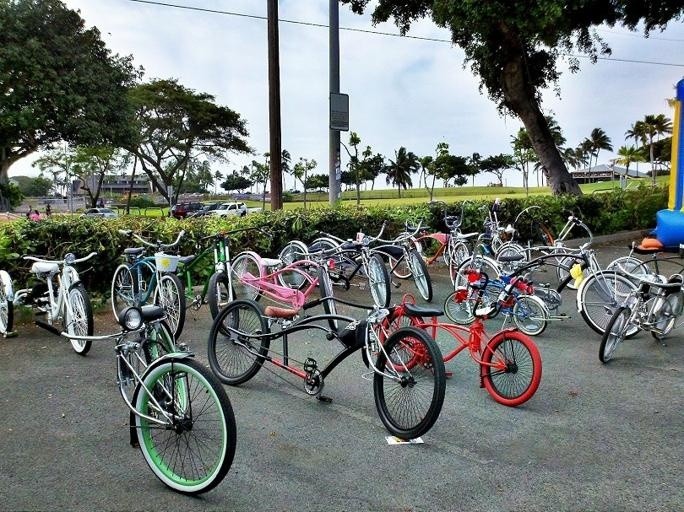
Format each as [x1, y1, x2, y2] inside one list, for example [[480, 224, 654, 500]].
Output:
[[205, 201, 248, 221], [168, 201, 203, 219], [186, 202, 221, 218]]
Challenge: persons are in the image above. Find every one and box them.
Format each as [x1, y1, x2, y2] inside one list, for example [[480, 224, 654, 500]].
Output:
[[26, 203, 51, 221], [234, 200, 237, 208], [171, 201, 201, 219], [240, 203, 246, 216]]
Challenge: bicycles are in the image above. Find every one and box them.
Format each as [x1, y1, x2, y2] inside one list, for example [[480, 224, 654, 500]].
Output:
[[232, 239, 353, 332], [169, 228, 271, 339], [206, 295, 445, 440], [39, 301, 236, 496], [111, 230, 187, 340]]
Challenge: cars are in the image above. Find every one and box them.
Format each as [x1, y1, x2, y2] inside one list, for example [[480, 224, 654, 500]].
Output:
[[78, 207, 119, 220]]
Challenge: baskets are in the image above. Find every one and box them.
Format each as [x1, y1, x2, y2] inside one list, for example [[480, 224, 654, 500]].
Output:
[[155, 250, 181, 272]]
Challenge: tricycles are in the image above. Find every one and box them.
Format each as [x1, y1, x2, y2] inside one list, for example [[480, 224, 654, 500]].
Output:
[[0, 251, 99, 355]]
